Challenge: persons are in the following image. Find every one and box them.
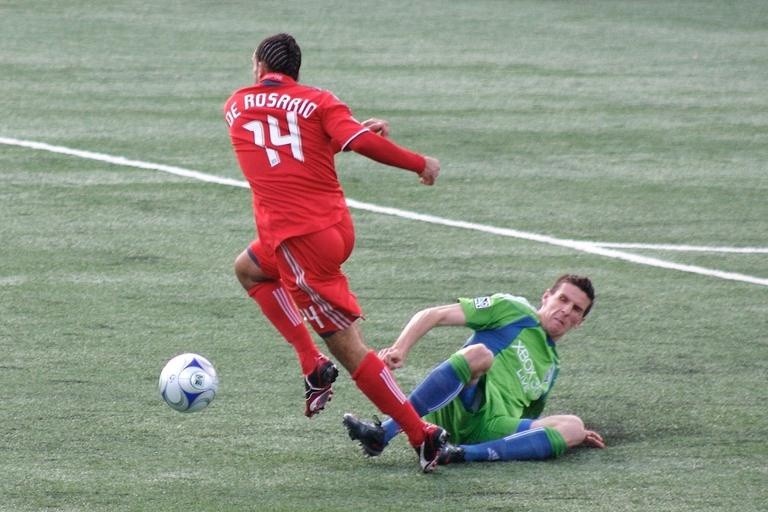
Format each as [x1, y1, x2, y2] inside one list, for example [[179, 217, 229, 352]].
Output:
[[222, 33, 452, 475], [341, 272, 608, 468]]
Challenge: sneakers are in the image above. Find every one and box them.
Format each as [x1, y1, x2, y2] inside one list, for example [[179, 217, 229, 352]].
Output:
[[343, 412, 388, 458], [304, 354, 341, 419], [414, 423, 450, 474], [439, 438, 463, 466]]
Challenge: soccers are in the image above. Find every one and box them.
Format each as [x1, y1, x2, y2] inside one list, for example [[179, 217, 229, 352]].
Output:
[[158, 353, 217, 413]]
